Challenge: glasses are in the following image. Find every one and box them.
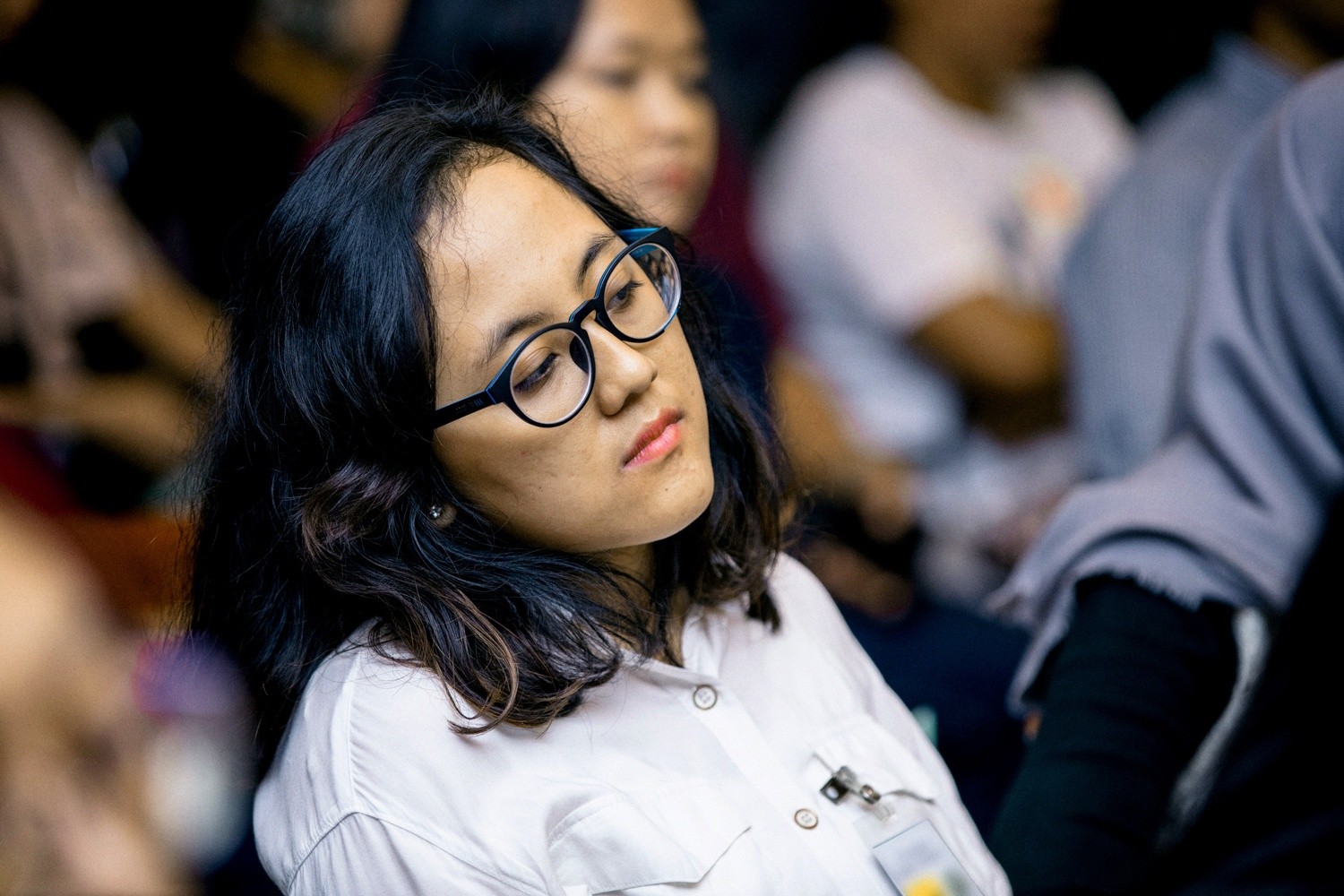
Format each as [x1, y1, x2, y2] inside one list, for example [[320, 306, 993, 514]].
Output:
[[422, 224, 684, 438]]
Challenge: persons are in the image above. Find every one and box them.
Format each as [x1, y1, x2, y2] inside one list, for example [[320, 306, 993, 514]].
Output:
[[189, 103, 1014, 896], [1, 3, 1344, 896]]
